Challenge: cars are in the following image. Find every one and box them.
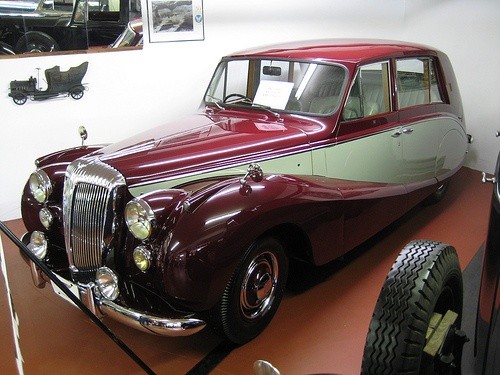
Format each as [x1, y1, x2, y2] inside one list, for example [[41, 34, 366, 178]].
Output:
[[21, 38, 474, 345], [0, 0, 130, 55], [106, 19, 144, 50]]
